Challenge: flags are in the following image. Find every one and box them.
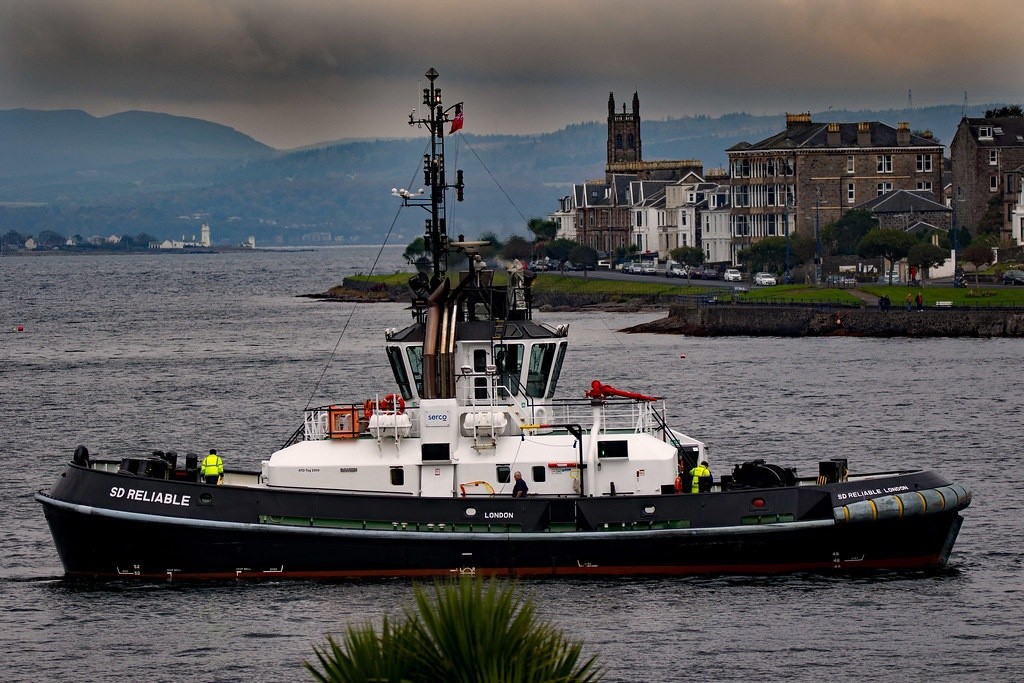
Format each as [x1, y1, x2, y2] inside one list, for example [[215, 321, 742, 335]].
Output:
[[449, 102, 464, 134]]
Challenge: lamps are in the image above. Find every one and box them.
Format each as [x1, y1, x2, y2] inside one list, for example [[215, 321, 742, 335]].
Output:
[[435, 88, 443, 105], [391, 328, 397, 337], [555, 325, 564, 336], [437, 154, 444, 170], [564, 323, 570, 336], [422, 88, 430, 103], [385, 329, 391, 339], [423, 153, 431, 169]]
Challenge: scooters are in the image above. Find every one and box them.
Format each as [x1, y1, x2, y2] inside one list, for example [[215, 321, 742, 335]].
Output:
[[956, 275, 969, 288]]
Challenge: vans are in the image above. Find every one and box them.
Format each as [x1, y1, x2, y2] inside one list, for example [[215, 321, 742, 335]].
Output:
[[723, 269, 742, 282], [884, 271, 899, 282]]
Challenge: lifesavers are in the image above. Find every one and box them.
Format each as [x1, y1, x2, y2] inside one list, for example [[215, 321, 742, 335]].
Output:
[[381, 394, 405, 416]]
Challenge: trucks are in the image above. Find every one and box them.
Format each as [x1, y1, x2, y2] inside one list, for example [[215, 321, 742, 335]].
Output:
[[665, 259, 684, 278]]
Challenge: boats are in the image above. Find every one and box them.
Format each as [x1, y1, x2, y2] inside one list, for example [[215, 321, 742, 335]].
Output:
[[34, 67, 973, 587]]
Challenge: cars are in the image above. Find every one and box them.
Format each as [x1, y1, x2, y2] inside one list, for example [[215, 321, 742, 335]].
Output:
[[529, 259, 560, 271], [1002, 269, 1024, 285], [825, 274, 844, 283], [621, 262, 656, 275], [563, 260, 584, 272], [684, 264, 719, 280], [753, 272, 776, 286]]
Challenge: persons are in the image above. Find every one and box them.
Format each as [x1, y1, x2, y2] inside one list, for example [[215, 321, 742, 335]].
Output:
[[954, 264, 968, 288], [200, 449, 224, 485], [496, 350, 505, 374], [511, 472, 528, 499], [879, 295, 891, 312], [905, 292, 923, 312], [689, 462, 714, 494]]
